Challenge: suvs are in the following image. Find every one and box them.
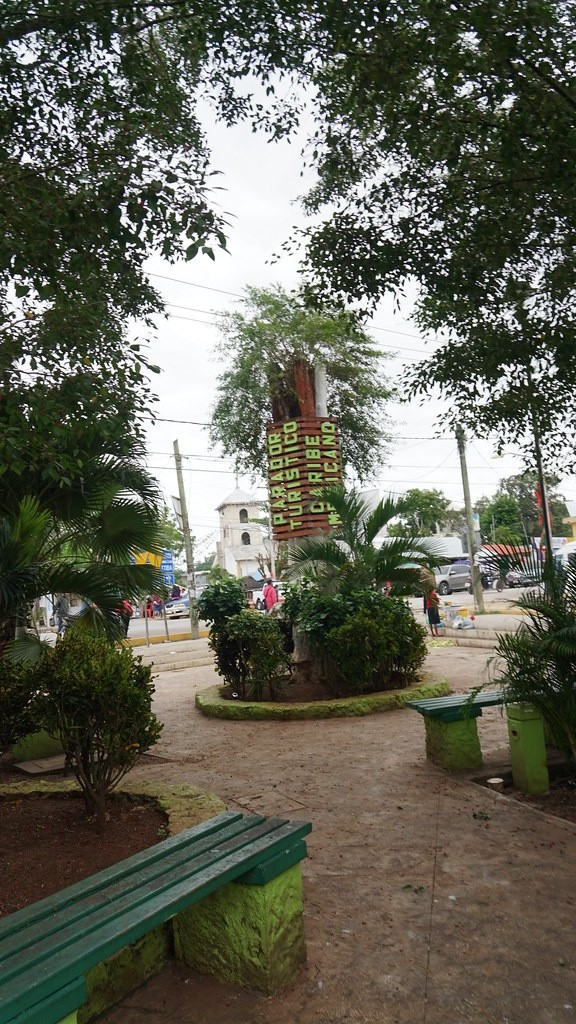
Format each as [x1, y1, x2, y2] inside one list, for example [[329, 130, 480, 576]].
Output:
[[431, 564, 472, 595]]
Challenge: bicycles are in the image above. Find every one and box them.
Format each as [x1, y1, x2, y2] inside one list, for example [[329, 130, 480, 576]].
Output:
[[493, 571, 509, 592]]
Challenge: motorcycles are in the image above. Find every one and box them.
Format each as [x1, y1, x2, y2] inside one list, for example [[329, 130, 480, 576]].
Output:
[[465, 573, 490, 594]]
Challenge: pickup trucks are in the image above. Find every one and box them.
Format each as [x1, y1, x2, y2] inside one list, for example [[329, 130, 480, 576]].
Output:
[[252, 581, 292, 610]]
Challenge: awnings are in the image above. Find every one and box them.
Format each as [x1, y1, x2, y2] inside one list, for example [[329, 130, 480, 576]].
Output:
[[484, 543, 531, 556]]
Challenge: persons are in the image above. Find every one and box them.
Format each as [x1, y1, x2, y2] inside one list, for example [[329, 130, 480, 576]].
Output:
[[147, 593, 164, 618], [264, 579, 278, 614], [114, 597, 133, 639], [423, 585, 443, 637], [180, 589, 188, 598], [53, 595, 69, 634]]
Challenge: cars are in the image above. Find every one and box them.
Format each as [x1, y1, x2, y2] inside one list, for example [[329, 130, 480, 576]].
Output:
[[164, 589, 204, 620], [554, 541, 576, 581], [508, 561, 544, 588]]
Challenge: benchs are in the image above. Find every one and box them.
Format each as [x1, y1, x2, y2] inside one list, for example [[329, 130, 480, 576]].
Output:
[[406, 690, 547, 774], [0, 810, 312, 1024]]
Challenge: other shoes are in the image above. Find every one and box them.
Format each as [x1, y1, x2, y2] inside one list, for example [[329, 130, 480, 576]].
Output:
[[432, 634, 435, 637], [124, 636, 131, 639], [435, 634, 444, 637]]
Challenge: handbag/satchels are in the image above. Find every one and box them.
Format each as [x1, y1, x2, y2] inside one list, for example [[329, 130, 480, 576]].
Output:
[[49, 615, 55, 627], [428, 596, 438, 607], [261, 599, 266, 608]]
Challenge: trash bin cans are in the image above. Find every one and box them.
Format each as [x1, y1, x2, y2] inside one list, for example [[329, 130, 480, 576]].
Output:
[[505, 704, 549, 796]]
